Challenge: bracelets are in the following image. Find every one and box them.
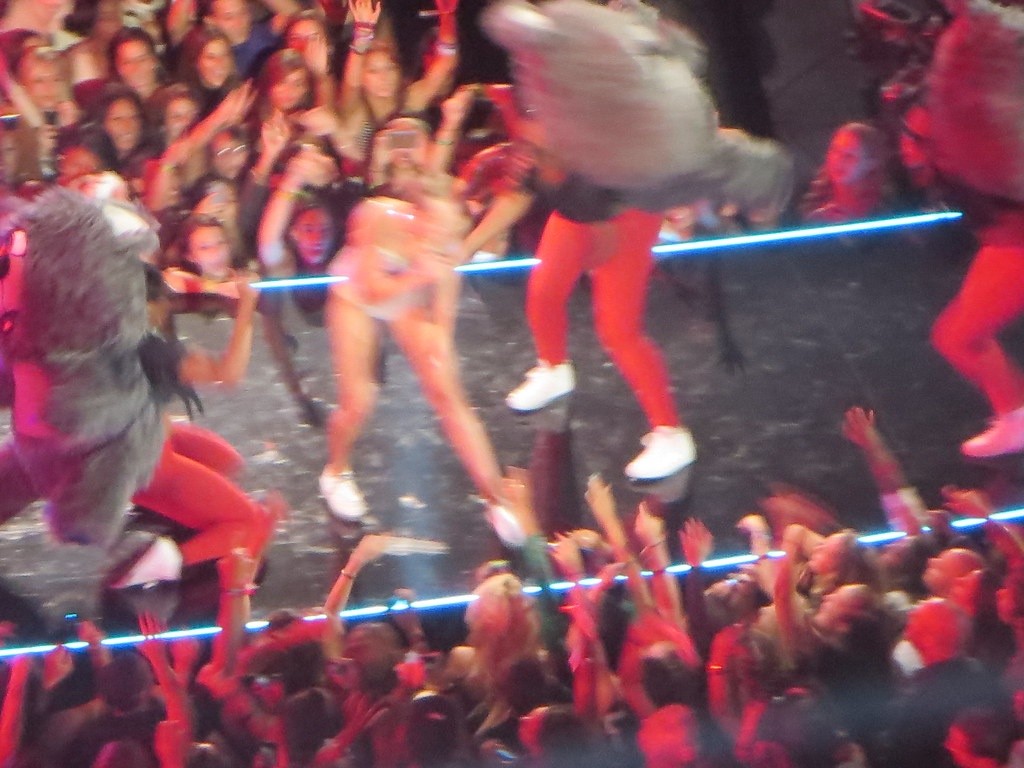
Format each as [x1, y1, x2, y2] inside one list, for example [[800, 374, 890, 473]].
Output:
[[273, 189, 296, 202], [348, 43, 370, 55], [354, 21, 376, 30], [640, 539, 669, 557], [653, 565, 669, 578], [222, 586, 256, 597], [436, 41, 460, 55], [340, 569, 356, 579], [352, 32, 374, 41]]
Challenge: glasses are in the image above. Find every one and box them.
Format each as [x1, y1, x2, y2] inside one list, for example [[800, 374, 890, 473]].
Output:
[[898, 116, 932, 146], [216, 143, 248, 159]]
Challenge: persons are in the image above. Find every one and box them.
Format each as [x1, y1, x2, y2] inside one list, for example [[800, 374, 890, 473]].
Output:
[[0, 0, 1023, 768]]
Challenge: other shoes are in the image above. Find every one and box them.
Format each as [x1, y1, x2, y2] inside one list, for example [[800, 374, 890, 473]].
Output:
[[106, 528, 183, 591], [624, 427, 698, 479], [484, 499, 528, 548], [959, 408, 1024, 457], [507, 360, 576, 412], [320, 463, 366, 520]]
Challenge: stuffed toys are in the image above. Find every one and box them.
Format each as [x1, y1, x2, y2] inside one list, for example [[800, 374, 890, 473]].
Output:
[[0, 187, 167, 553], [479, 0, 798, 224]]
